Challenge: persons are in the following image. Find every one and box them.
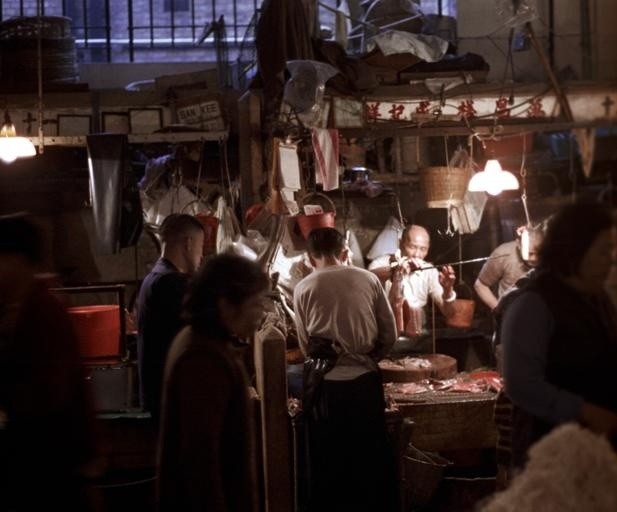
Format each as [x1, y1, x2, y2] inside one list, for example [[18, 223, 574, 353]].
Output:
[[366, 224, 458, 338], [0, 217, 108, 511], [473, 220, 545, 312], [132, 213, 206, 420], [294, 227, 402, 511], [156, 253, 270, 512], [503, 198, 617, 476]]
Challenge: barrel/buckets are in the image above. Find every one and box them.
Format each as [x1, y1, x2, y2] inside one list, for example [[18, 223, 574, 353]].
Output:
[[297, 193, 337, 243], [67, 304, 121, 358], [446, 282, 477, 328], [189, 201, 221, 256], [86, 368, 132, 410]]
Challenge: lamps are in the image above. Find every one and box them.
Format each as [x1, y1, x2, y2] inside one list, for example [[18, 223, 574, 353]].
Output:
[[0, 109, 37, 164], [467, 139, 522, 200]]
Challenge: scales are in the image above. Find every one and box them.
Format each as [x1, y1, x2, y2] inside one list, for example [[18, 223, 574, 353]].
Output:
[[410, 253, 511, 276]]
